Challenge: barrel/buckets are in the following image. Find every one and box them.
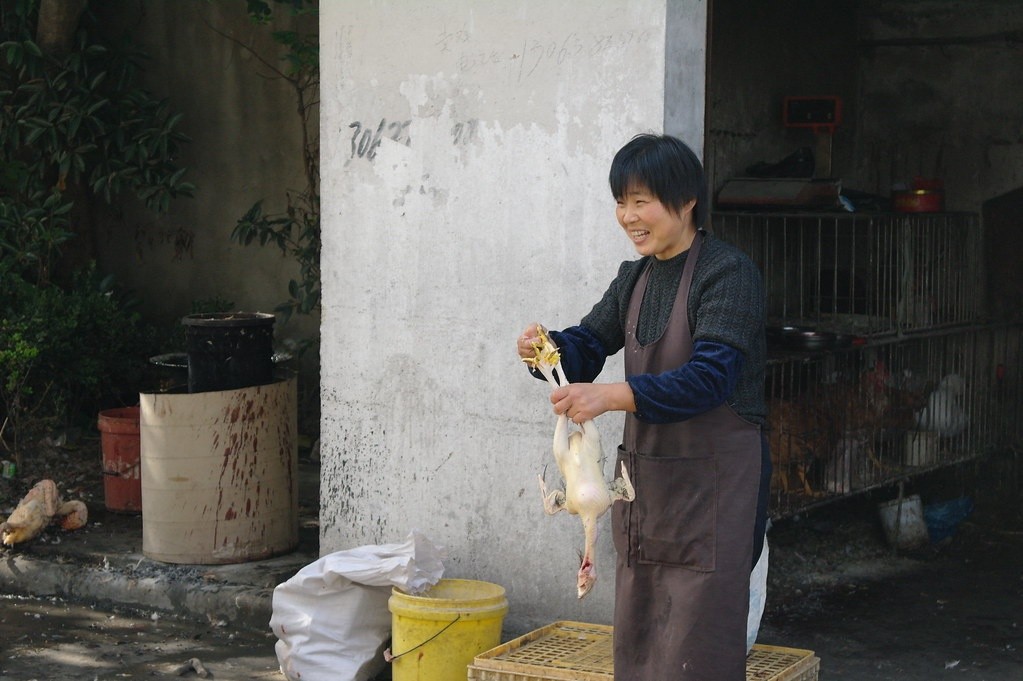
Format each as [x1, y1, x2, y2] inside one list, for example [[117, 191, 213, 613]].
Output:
[[150, 351, 187, 394], [96, 407, 142, 513], [383, 579, 508, 681], [183, 312, 275, 393]]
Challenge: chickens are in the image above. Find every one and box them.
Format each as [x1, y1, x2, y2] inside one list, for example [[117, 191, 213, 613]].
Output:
[[762, 358, 968, 502], [521, 323, 636, 600]]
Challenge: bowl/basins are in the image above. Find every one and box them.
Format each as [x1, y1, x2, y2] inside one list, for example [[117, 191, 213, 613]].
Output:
[[766, 326, 816, 342], [837, 334, 856, 346], [890, 187, 947, 213], [787, 330, 837, 350]]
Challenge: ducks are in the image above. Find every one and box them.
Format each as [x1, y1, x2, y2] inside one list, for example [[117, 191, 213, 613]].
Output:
[[0, 479, 88, 550]]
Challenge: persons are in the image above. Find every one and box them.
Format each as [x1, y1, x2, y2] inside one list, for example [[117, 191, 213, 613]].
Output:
[[515, 130, 771, 681]]
